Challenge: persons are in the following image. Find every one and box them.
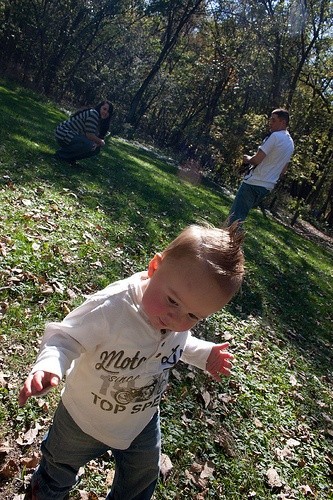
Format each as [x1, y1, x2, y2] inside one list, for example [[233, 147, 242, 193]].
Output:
[[228, 108, 295, 228], [19, 219, 246, 500], [51, 100, 114, 163]]
